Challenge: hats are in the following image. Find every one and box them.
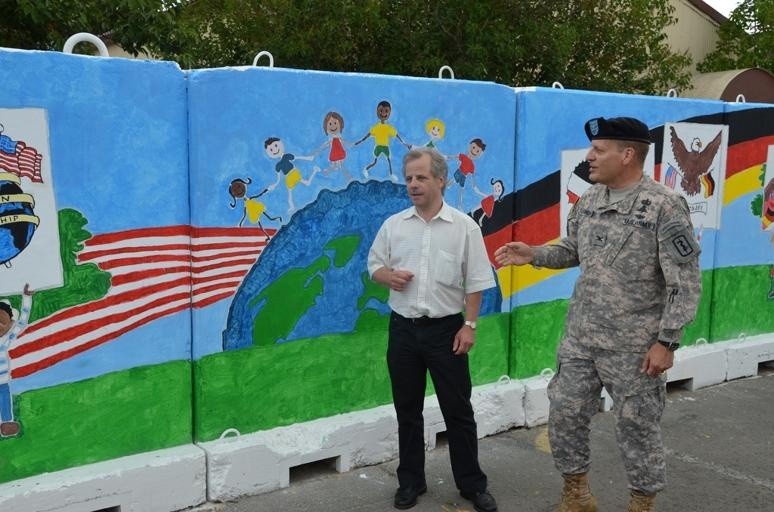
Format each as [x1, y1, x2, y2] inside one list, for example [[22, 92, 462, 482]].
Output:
[[583, 117, 653, 143]]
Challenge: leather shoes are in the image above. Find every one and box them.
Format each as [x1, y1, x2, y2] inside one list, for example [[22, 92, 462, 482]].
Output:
[[459, 488, 497, 512], [391, 482, 427, 509]]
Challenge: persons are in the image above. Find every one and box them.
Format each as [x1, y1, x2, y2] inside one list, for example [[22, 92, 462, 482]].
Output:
[[366, 147, 497, 511], [494, 115, 701, 512]]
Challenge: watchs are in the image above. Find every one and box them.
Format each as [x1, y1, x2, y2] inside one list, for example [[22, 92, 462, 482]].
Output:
[[658, 340, 680, 350], [464, 321, 477, 329]]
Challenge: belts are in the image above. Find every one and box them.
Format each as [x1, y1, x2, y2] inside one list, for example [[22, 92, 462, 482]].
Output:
[[391, 311, 463, 326]]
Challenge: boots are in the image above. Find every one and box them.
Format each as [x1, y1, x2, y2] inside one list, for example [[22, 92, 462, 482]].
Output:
[[627, 488, 659, 512], [551, 469, 600, 512]]
